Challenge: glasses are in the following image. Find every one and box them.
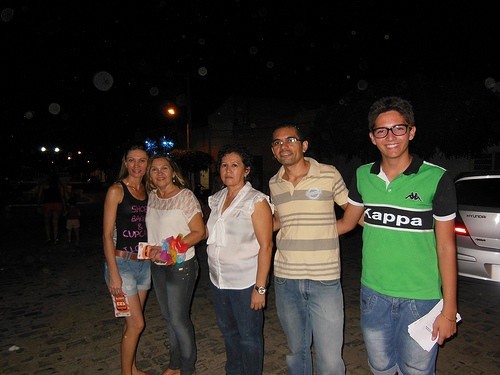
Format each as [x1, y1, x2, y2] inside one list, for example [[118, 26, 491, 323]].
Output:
[[272, 137, 303, 146], [373, 124, 408, 138]]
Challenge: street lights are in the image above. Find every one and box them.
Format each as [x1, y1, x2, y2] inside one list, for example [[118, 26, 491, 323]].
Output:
[[164, 107, 193, 188]]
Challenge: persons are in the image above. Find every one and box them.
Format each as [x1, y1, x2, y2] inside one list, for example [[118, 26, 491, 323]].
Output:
[[269, 121, 364, 375], [204, 145, 272, 375], [37, 165, 64, 246], [336, 96, 457, 375], [103, 142, 151, 375], [62, 196, 81, 247], [145, 152, 205, 375]]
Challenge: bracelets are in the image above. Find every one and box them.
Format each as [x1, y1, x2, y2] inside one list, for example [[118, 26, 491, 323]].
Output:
[[441, 311, 456, 322], [160, 234, 188, 266]]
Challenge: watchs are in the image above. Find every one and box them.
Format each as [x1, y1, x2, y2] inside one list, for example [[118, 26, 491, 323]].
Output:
[[254, 284, 266, 295]]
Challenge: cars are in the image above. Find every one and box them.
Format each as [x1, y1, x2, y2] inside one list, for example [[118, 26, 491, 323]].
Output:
[[452, 174, 500, 284]]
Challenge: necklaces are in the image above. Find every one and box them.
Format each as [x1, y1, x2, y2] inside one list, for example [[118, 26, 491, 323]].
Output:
[[126, 177, 143, 195]]
